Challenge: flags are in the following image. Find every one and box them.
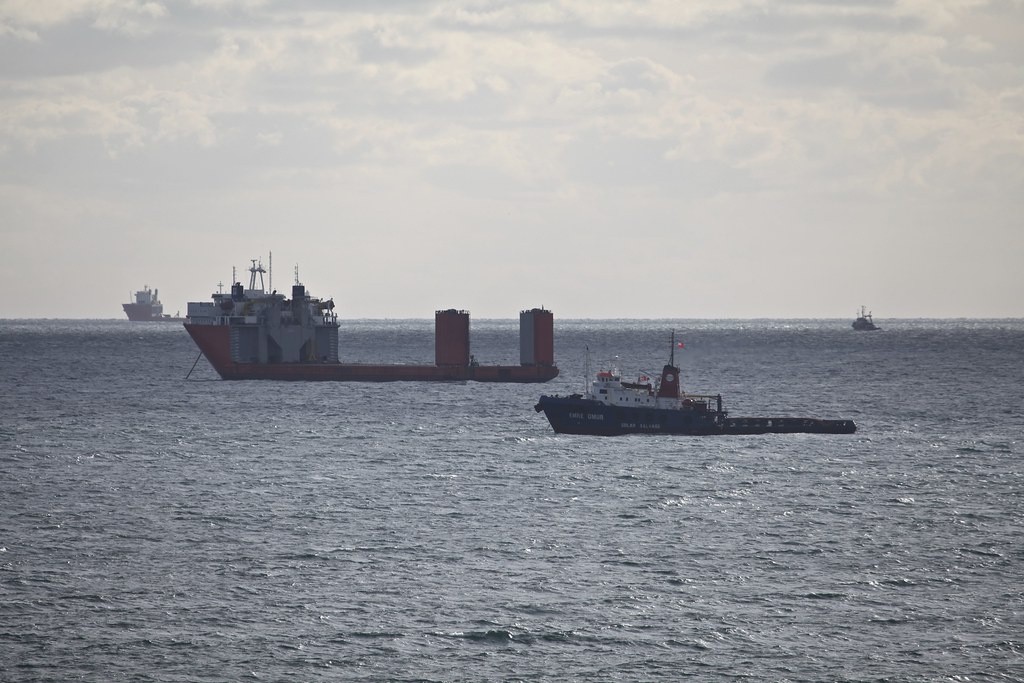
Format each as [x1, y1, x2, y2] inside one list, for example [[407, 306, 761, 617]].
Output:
[[678, 341, 684, 349]]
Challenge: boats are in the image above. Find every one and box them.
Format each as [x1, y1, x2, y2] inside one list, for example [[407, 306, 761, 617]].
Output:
[[182, 250, 560, 384], [120, 285, 184, 322], [535, 329, 859, 435], [851, 305, 881, 331]]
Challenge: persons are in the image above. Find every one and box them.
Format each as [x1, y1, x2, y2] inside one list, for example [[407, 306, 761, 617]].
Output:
[[469, 354, 479, 366]]
